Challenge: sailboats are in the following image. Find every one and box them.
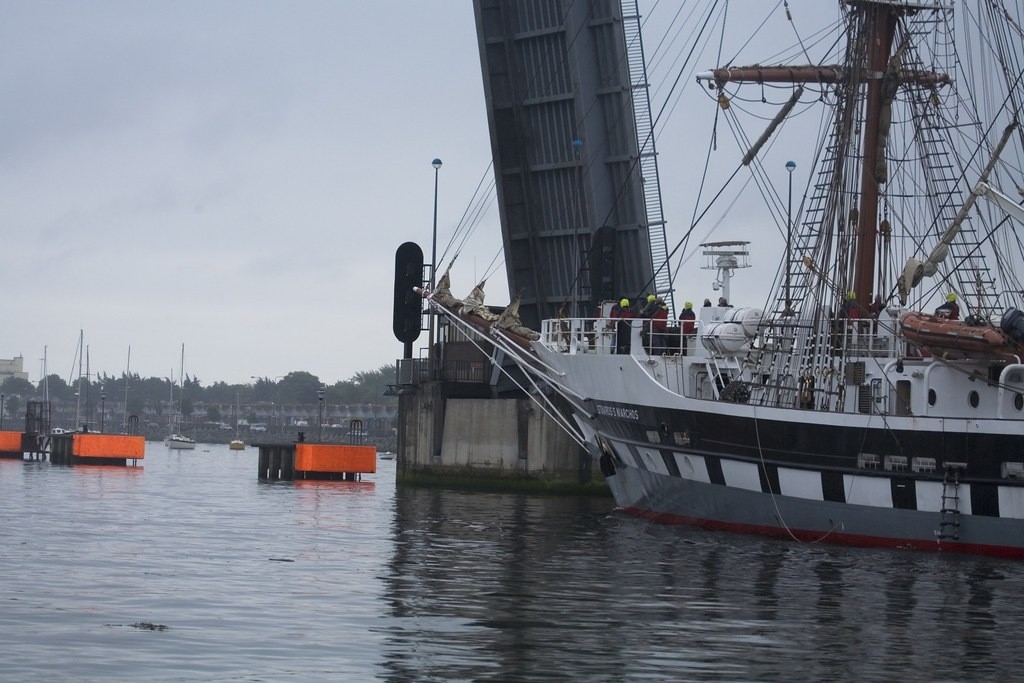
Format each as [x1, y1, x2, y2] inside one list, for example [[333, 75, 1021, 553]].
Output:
[[412, 0, 1024, 561]]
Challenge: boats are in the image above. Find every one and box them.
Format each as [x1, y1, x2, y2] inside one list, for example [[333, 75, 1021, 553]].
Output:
[[163, 432, 196, 451], [227, 438, 248, 451]]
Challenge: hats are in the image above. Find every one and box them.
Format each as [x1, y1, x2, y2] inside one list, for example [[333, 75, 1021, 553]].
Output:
[[656, 297, 664, 303], [720, 298, 726, 305], [875, 295, 881, 299]]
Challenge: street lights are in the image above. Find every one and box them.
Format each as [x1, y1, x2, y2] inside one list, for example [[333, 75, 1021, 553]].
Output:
[[426, 156, 442, 380]]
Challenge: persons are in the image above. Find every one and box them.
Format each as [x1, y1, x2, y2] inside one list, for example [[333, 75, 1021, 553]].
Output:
[[935, 293, 959, 320], [777, 301, 796, 348], [835, 291, 886, 337], [595, 294, 732, 356]]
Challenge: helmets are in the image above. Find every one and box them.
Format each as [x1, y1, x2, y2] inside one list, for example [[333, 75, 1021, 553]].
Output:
[[946, 293, 957, 302], [647, 295, 655, 302], [685, 301, 692, 309], [846, 291, 855, 300], [620, 299, 629, 308]]
